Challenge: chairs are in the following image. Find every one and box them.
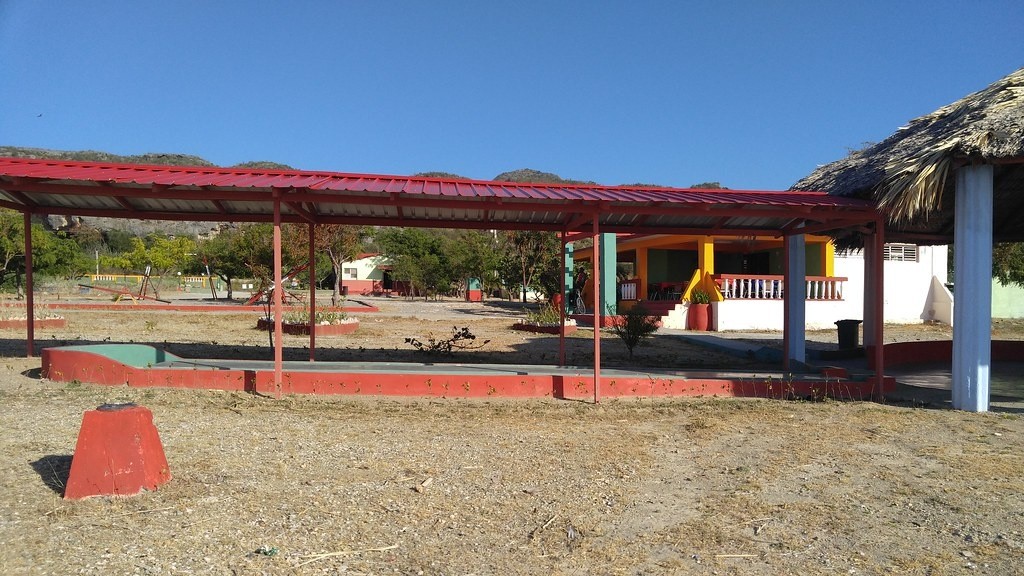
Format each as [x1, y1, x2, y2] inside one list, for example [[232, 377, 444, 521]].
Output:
[[648, 280, 689, 302]]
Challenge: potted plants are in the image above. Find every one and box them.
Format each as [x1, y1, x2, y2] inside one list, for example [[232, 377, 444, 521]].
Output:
[[514, 302, 577, 338], [260, 302, 360, 336], [687, 288, 714, 332]]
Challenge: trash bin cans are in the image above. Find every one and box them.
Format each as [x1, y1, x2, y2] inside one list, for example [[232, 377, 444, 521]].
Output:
[[834, 319, 863, 349]]
